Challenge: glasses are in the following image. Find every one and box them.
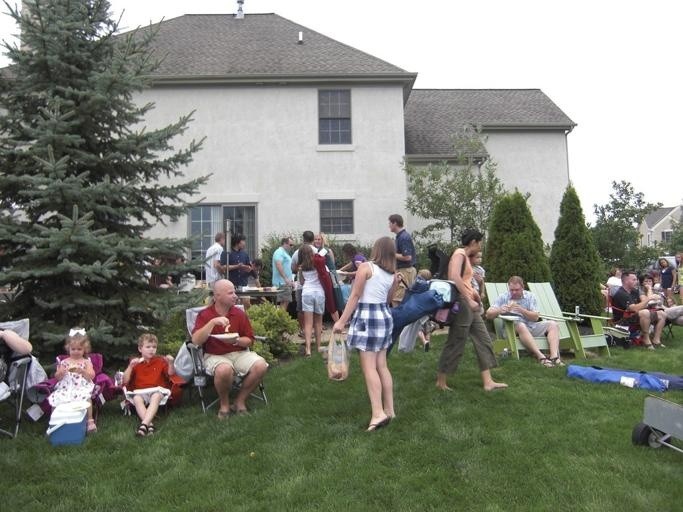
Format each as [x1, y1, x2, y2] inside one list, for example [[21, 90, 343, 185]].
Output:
[[287, 244, 295, 246]]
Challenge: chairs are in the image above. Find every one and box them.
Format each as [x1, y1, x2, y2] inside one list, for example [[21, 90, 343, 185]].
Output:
[[607, 284, 644, 347], [653, 290, 675, 341], [483, 283, 582, 365], [526, 281, 612, 360], [0, 318, 33, 439], [186, 304, 268, 415], [56, 352, 104, 427], [124, 357, 185, 419]]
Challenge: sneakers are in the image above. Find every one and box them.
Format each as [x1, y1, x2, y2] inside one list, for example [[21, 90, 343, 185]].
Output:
[[424, 341, 430, 352]]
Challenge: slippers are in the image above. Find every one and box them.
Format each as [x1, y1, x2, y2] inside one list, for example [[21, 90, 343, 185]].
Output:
[[217, 410, 231, 422], [651, 337, 668, 348], [231, 404, 248, 414], [365, 416, 390, 433], [639, 337, 656, 352]]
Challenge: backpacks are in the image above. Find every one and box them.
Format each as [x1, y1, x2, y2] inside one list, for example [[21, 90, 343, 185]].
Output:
[[426, 279, 461, 329]]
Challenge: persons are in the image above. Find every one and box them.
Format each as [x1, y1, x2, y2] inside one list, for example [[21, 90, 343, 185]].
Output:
[[338, 243, 369, 282], [414, 268, 432, 354], [612, 272, 665, 351], [389, 215, 417, 308], [291, 231, 346, 319], [484, 276, 567, 367], [51, 326, 98, 436], [657, 258, 676, 304], [652, 277, 665, 298], [297, 244, 329, 358], [0, 327, 33, 383], [219, 233, 253, 286], [272, 236, 295, 312], [120, 334, 176, 437], [675, 253, 682, 305], [314, 231, 344, 309], [247, 259, 268, 305], [160, 257, 196, 290], [191, 278, 269, 421], [436, 229, 509, 393], [332, 237, 398, 434], [205, 232, 225, 305], [470, 250, 490, 320], [608, 267, 623, 303], [638, 274, 682, 326]]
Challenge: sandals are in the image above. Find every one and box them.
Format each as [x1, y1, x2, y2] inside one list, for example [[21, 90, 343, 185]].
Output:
[[135, 423, 148, 438], [87, 421, 97, 431], [539, 358, 555, 368], [146, 425, 156, 436], [549, 356, 566, 367]]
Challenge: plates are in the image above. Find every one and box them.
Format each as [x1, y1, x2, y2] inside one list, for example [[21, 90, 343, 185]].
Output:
[[211, 332, 238, 343]]
